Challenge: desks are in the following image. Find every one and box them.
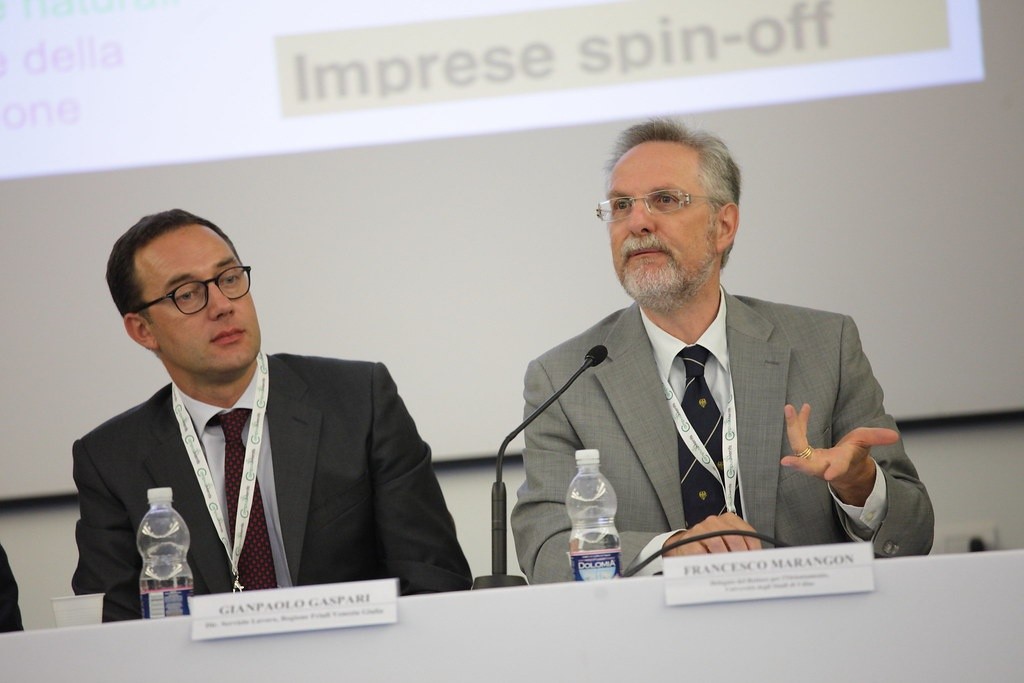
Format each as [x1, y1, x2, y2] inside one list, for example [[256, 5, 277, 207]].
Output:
[[0, 544, 1024, 683]]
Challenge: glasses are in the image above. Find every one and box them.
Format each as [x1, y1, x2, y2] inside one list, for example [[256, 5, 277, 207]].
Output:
[[596, 189, 727, 223], [136, 266, 251, 315]]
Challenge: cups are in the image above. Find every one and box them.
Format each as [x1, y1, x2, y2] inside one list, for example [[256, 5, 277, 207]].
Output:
[[50, 593, 106, 629]]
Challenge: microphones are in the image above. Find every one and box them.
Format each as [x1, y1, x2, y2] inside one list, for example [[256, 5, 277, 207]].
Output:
[[471, 345, 609, 590]]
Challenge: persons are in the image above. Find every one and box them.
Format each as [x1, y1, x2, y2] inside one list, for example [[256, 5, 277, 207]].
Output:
[[71, 209, 474, 622], [510, 116, 935, 586]]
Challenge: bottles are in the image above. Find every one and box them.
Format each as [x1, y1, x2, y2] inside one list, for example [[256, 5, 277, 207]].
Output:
[[136, 487, 194, 619], [566, 448, 623, 581]]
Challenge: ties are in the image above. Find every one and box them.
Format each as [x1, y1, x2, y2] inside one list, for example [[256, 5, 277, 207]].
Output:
[[675, 344, 743, 530], [206, 408, 278, 592]]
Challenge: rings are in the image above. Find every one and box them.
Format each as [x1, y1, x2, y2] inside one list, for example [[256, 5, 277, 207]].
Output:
[[794, 445, 814, 460]]
